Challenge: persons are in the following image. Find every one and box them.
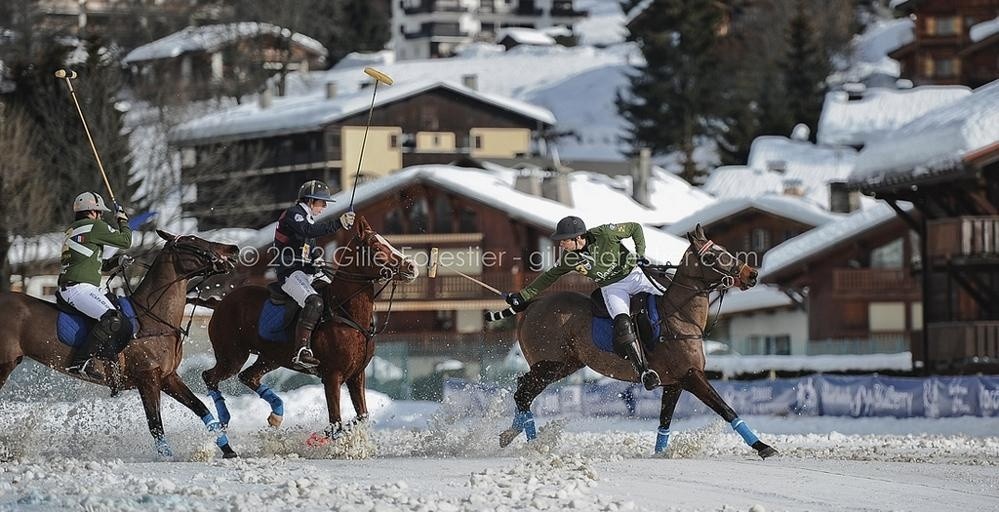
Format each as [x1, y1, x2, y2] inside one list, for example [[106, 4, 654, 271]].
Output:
[[56, 191, 132, 383], [273, 178, 356, 371], [506, 215, 672, 391]]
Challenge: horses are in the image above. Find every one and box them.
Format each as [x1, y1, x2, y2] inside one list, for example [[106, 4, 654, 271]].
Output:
[[484, 223, 780, 461], [184, 215, 419, 441], [0, 229, 240, 463]]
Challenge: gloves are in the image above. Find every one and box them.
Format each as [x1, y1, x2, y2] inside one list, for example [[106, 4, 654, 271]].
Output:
[[340, 210, 356, 230], [113, 198, 129, 224], [636, 257, 651, 267], [506, 292, 526, 306]]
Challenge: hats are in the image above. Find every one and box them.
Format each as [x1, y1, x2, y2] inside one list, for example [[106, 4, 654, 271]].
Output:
[[550, 216, 587, 240]]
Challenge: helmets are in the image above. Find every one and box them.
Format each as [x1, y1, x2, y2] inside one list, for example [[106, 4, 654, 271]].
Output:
[[73, 191, 112, 213], [298, 180, 337, 203]]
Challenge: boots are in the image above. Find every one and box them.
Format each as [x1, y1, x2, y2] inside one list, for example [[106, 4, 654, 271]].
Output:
[[289, 307, 324, 370], [618, 324, 660, 390], [70, 316, 123, 381]]
